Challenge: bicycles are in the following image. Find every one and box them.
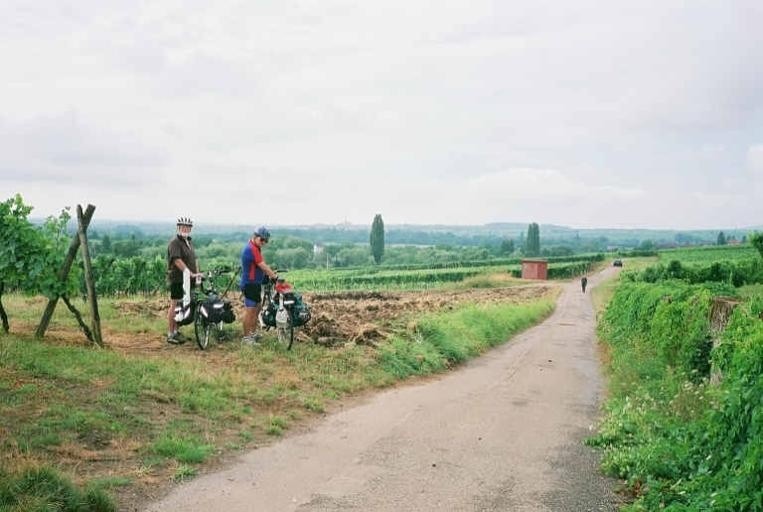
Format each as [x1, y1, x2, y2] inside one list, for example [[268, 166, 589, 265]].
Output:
[[193, 266, 229, 351], [260, 269, 296, 349]]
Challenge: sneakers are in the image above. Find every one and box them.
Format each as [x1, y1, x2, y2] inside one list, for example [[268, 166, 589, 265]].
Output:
[[167, 331, 192, 345], [242, 332, 263, 346]]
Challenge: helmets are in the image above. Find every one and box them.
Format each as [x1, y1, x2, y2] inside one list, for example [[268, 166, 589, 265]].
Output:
[[254, 225, 270, 242], [176, 215, 192, 225]]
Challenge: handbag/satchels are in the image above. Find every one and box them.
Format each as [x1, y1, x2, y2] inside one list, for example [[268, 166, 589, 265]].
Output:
[[174, 298, 234, 325], [263, 292, 311, 329]]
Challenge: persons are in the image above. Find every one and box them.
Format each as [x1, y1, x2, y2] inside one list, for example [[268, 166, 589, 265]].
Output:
[[581, 275, 587, 293], [240, 225, 277, 347], [166, 215, 203, 344]]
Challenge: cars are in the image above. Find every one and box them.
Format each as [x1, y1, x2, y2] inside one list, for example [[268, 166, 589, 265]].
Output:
[[614, 260, 622, 267]]
